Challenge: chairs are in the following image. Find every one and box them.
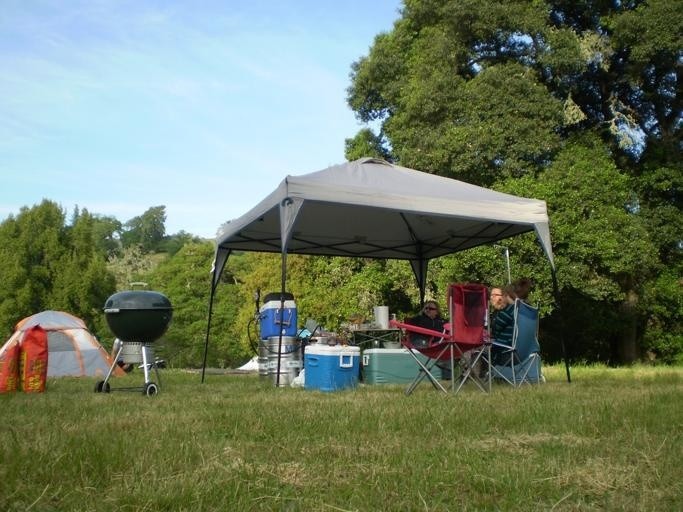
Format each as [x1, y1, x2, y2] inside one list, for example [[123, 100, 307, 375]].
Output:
[[389, 281, 543, 397]]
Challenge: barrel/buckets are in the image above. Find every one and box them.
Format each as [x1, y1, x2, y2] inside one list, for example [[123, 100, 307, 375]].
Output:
[[267, 336, 299, 387], [258, 341, 267, 385]]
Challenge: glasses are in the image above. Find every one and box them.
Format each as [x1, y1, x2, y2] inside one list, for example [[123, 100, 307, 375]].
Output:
[[425, 307, 437, 311]]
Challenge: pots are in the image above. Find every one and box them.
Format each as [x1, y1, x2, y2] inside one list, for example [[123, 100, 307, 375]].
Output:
[[100, 281, 175, 342]]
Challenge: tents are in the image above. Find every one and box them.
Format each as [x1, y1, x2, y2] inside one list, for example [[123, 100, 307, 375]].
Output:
[[0, 309, 129, 380]]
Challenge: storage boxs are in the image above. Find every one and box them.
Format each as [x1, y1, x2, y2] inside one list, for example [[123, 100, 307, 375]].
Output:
[[256, 291, 447, 393]]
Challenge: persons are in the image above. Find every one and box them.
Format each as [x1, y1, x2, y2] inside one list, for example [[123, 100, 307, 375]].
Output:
[[408, 299, 455, 381], [490, 276, 533, 367], [457, 284, 504, 385]]
